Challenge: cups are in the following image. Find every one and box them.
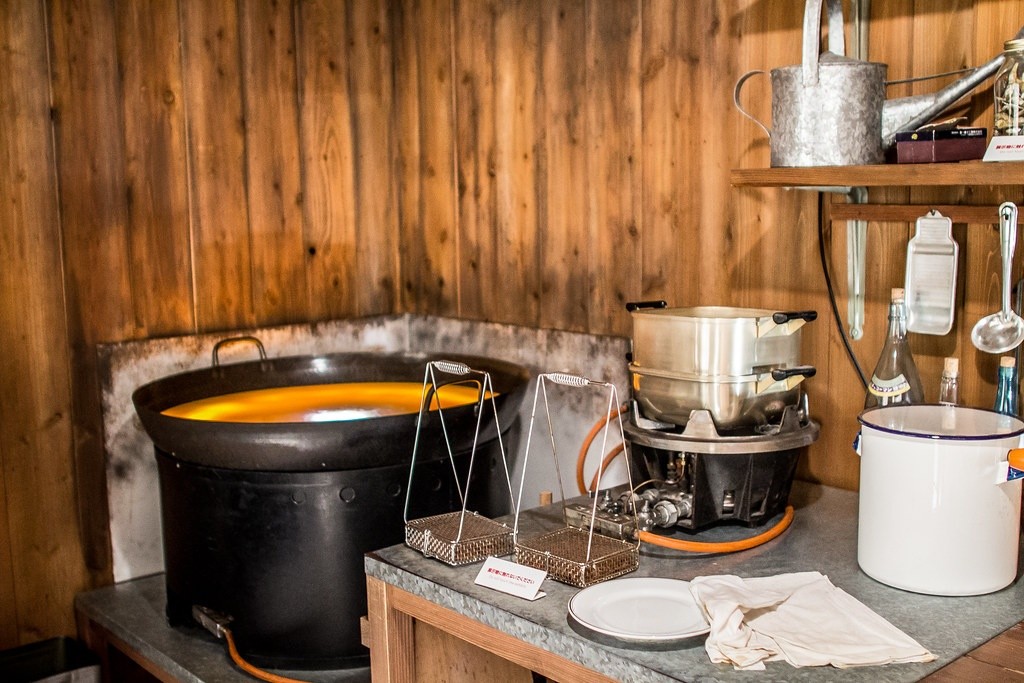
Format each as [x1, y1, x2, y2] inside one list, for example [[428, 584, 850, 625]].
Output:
[[858, 405, 1024, 597]]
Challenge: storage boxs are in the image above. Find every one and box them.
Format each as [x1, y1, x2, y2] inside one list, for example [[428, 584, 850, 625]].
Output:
[[896, 128, 988, 163]]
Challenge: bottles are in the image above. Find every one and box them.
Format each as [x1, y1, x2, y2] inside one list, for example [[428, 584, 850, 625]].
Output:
[[939, 374, 958, 407], [994, 366, 1019, 418], [864, 301, 924, 412]]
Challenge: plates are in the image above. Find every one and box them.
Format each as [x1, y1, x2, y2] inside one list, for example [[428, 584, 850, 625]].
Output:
[[568, 577, 711, 641]]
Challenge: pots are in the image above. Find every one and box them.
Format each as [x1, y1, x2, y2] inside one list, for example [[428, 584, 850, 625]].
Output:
[[628, 297, 814, 432], [131, 336, 528, 475]]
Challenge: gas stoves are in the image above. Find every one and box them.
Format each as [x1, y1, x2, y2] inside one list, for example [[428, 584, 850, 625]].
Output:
[[620, 416, 820, 534], [155, 445, 498, 683]]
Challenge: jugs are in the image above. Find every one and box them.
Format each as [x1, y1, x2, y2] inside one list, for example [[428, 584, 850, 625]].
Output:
[[733, 0, 1005, 168]]
